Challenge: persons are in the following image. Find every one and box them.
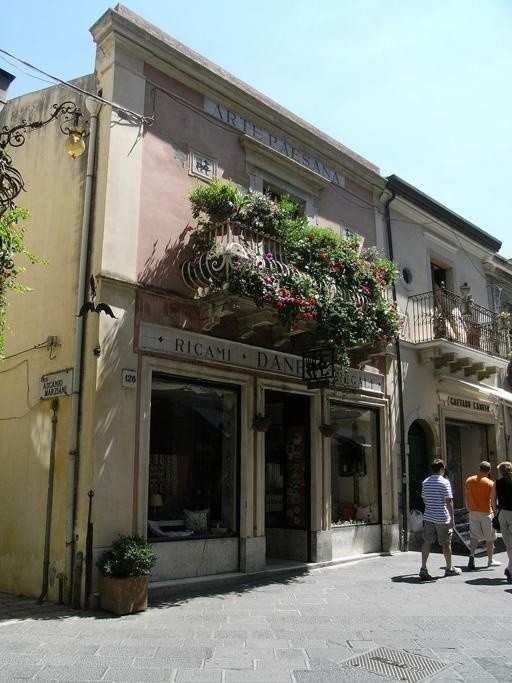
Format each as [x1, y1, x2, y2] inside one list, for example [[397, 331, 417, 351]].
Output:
[[491, 461, 512, 584], [464, 460, 501, 570], [418, 458, 463, 580]]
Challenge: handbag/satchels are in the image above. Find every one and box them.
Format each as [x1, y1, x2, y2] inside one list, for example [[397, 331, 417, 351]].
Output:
[[491, 516, 500, 531]]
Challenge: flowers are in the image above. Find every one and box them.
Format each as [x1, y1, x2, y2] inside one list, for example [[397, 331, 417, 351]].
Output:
[[181, 186, 407, 370]]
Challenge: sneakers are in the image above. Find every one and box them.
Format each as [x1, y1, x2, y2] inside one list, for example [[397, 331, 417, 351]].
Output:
[[504, 568, 512, 583], [488, 559, 501, 567], [419, 568, 431, 580], [468, 554, 475, 570], [445, 567, 462, 576]]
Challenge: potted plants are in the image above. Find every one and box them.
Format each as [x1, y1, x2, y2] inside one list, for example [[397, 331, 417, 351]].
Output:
[[253, 410, 272, 432], [95, 533, 160, 615], [319, 423, 337, 437]]
